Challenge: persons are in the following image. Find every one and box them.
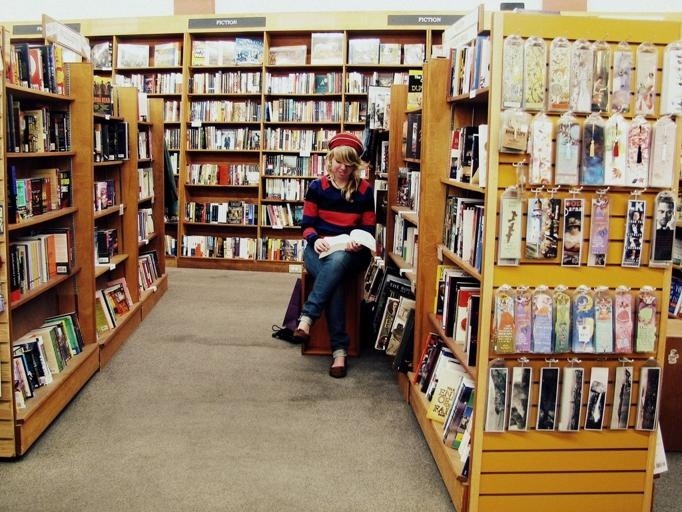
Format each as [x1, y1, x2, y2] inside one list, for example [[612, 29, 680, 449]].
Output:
[[626, 210, 642, 265], [563, 218, 579, 252], [269, 135, 377, 379], [656, 196, 674, 232]]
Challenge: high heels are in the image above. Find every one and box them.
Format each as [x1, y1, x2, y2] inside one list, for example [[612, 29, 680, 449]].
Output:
[[287, 328, 310, 352]]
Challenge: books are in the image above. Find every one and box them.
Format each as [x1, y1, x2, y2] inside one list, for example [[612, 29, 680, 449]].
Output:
[[137, 72, 182, 297], [264, 71, 343, 124], [185, 126, 262, 185], [344, 70, 394, 152], [264, 127, 338, 177], [371, 34, 488, 480], [95, 276, 134, 333], [7, 163, 73, 301], [93, 175, 119, 267], [12, 311, 85, 412], [259, 178, 314, 261], [92, 76, 131, 162], [190, 70, 262, 122], [4, 29, 72, 153], [91, 31, 379, 67], [183, 201, 257, 259]]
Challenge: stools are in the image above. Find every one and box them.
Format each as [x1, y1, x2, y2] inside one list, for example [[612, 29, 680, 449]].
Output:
[[300, 264, 364, 359]]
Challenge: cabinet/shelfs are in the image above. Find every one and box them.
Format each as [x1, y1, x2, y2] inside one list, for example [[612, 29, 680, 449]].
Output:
[[91, 19, 442, 274], [1, 26, 168, 461], [366, 4, 681, 512]]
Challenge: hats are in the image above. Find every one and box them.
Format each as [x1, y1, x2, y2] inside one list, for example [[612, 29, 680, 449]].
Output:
[[328, 133, 364, 157]]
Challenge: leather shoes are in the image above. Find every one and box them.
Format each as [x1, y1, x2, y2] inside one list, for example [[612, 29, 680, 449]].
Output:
[[329, 356, 348, 379]]
